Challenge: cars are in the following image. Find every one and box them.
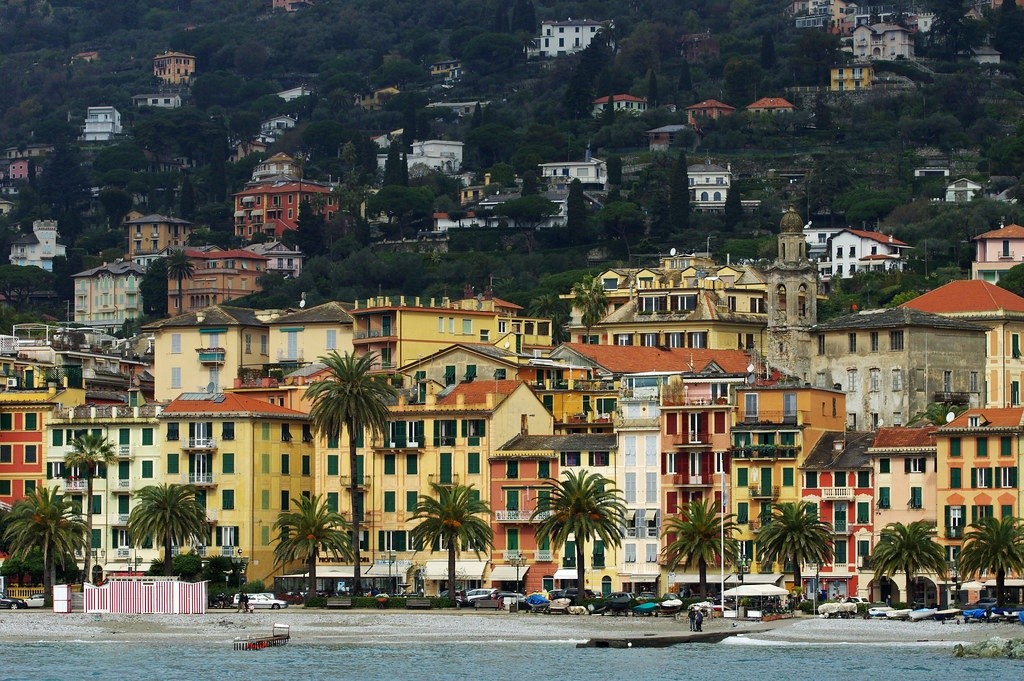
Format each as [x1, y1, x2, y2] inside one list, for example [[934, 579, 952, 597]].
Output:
[[548, 588, 596, 600], [662, 593, 678, 600], [436, 587, 527, 603], [0, 591, 26, 609], [690, 592, 734, 603], [977, 598, 998, 610], [23, 593, 45, 609], [842, 597, 871, 606], [640, 592, 656, 599], [606, 593, 634, 599]]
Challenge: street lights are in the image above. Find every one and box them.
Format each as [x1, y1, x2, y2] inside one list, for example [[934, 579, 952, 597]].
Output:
[[509, 558, 527, 614], [381, 550, 397, 597], [735, 557, 752, 584]]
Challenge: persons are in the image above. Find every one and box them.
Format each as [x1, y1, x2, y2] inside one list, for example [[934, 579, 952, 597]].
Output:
[[886, 595, 892, 606], [1003, 592, 1013, 606], [216, 593, 226, 609], [688, 607, 703, 632], [234, 590, 255, 614]]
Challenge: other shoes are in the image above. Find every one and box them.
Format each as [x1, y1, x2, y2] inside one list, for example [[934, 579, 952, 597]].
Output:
[[690, 630, 692, 631], [700, 630, 702, 632], [696, 630, 698, 631], [693, 630, 696, 631]]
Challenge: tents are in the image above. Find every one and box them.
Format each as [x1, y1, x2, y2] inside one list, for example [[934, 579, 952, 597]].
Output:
[[725, 582, 790, 617]]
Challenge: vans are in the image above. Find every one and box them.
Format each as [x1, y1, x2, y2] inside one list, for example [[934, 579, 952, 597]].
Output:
[[233, 593, 289, 609]]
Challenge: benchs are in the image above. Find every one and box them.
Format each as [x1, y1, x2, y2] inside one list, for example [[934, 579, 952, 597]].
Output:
[[405, 599, 431, 610], [327, 599, 351, 609], [475, 600, 499, 611]]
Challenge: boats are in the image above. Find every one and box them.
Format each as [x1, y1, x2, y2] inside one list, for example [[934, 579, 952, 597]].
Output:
[[934, 608, 961, 621], [634, 602, 656, 613], [907, 607, 937, 619], [962, 607, 1024, 623], [526, 593, 587, 616], [660, 598, 683, 612], [605, 595, 630, 611], [867, 607, 895, 616], [818, 602, 857, 615], [885, 608, 913, 619]]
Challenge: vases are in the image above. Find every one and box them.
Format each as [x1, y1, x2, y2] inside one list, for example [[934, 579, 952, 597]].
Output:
[[763, 614, 792, 621]]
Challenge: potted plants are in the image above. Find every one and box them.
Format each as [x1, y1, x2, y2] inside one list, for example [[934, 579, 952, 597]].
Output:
[[791, 586, 803, 617], [738, 597, 750, 618]]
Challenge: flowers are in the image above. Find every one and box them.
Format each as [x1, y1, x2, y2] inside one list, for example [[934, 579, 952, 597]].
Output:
[[762, 609, 791, 616]]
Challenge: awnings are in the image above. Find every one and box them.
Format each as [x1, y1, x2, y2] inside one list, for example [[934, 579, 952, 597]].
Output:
[[985, 579, 1024, 587], [554, 569, 580, 580], [420, 562, 487, 581], [623, 509, 657, 521], [489, 564, 531, 582], [945, 582, 986, 592], [306, 563, 413, 578], [672, 574, 784, 583], [627, 573, 661, 582]]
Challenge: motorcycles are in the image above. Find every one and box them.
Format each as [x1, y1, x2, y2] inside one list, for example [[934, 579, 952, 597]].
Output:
[[215, 593, 226, 609]]
